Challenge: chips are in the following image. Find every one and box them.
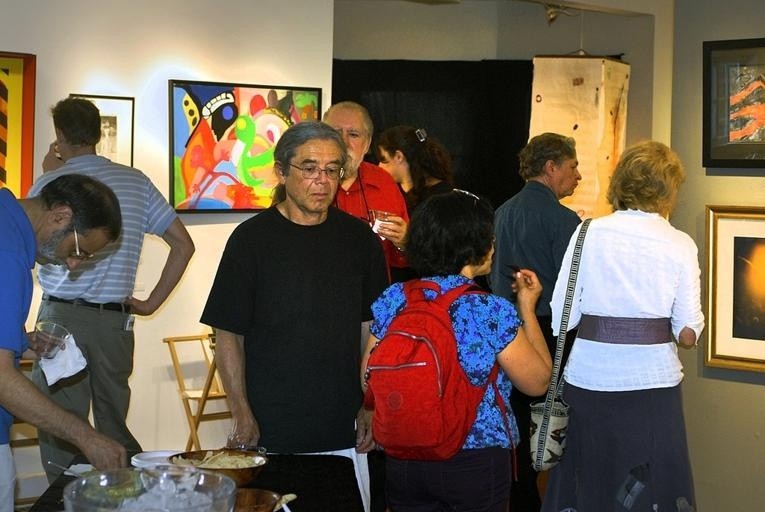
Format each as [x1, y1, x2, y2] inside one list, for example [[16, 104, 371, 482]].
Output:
[[172, 450, 260, 470]]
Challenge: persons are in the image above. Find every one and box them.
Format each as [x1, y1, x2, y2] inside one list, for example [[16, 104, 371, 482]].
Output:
[[200, 103, 704, 512], [0, 98, 195, 512]]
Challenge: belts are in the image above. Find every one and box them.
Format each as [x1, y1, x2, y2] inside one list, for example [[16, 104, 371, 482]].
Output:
[[42, 294, 131, 312]]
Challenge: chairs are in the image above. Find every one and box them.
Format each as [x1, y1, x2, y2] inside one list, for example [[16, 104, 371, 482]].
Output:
[[161, 333, 235, 453], [10, 359, 55, 511]]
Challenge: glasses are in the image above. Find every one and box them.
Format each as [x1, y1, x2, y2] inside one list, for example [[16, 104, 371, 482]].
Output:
[[70, 224, 95, 261], [288, 164, 345, 180]]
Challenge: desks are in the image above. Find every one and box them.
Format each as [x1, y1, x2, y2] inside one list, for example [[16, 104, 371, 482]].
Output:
[[26, 452, 367, 512]]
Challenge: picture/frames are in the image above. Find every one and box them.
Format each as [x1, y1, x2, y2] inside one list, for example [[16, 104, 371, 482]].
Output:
[[700, 36, 764, 168], [165, 77, 327, 215], [0, 50, 37, 200], [68, 92, 137, 184], [699, 201, 764, 373]]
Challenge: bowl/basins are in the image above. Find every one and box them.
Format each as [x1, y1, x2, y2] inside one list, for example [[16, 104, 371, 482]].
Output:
[[169, 449, 269, 488], [231, 488, 284, 512], [64, 467, 236, 512]]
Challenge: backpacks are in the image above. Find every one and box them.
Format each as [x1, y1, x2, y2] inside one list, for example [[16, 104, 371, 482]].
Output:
[[364, 279, 499, 461]]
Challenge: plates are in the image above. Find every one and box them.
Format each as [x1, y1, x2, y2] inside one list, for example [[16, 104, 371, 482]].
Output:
[[130, 450, 177, 468]]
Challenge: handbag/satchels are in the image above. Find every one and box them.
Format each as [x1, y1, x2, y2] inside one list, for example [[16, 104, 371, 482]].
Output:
[[529, 399, 570, 472]]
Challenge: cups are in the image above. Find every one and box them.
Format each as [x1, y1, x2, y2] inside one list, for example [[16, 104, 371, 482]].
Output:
[[367, 209, 397, 232], [35, 320, 69, 361]]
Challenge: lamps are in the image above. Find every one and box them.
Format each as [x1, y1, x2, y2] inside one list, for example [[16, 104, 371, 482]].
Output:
[[524, 5, 633, 224]]
